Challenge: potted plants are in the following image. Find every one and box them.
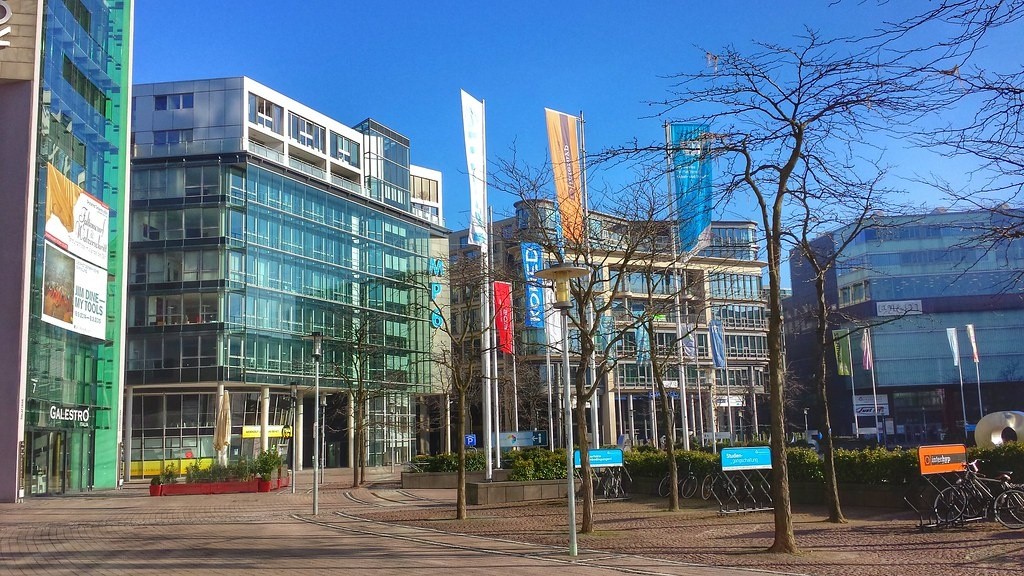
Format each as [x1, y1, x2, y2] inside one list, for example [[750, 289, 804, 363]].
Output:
[[149, 475, 161, 496], [258, 449, 272, 492]]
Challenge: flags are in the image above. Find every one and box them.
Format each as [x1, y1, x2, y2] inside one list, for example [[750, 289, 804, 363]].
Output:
[[460, 88, 486, 249], [632, 310, 651, 362], [669, 124, 713, 263], [832, 329, 851, 376], [520, 241, 545, 329], [965, 324, 979, 362], [946, 328, 959, 366], [599, 316, 616, 368], [494, 281, 513, 355], [859, 327, 873, 371], [680, 323, 696, 356], [708, 320, 726, 367], [544, 107, 585, 246]]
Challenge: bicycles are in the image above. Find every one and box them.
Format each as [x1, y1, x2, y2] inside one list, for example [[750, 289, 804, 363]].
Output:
[[933, 459, 1024, 533], [700, 459, 750, 503], [603, 460, 631, 498], [657, 458, 698, 499]]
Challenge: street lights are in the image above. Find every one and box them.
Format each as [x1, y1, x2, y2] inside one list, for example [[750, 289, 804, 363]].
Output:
[[307, 331, 324, 515], [537, 261, 594, 555], [290, 381, 297, 494]]
